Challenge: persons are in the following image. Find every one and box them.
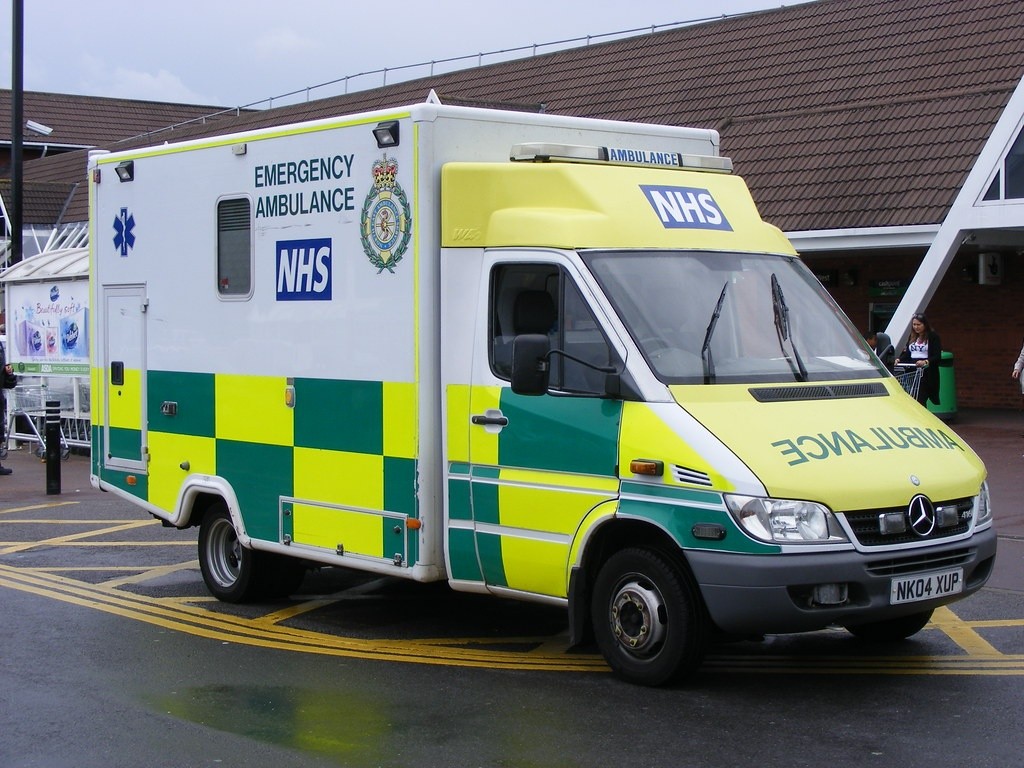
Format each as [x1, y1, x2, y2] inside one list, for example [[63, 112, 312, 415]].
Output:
[[894, 313, 942, 409], [864, 330, 877, 356], [0, 341, 18, 476], [1012, 346, 1024, 395]]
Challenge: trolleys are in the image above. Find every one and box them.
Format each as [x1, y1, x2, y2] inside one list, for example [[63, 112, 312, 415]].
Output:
[[0, 382, 91, 461], [888, 363, 929, 401]]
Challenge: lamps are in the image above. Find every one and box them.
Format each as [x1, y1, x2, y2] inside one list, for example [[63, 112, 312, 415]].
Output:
[[372, 122, 400, 145], [115, 161, 133, 180]]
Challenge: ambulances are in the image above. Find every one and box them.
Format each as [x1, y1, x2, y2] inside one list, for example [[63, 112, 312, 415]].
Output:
[[84, 89, 1000, 688]]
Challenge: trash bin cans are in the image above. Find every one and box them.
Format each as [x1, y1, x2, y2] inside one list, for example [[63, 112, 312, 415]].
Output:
[[925, 352, 958, 421]]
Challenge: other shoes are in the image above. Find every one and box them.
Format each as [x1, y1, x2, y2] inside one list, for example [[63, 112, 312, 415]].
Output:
[[0, 467, 12, 475]]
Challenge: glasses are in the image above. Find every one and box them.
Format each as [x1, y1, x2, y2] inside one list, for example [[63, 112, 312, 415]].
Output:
[[912, 314, 924, 318]]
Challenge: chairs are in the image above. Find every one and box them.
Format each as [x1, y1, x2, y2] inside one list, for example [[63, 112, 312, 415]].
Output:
[[515, 291, 556, 337]]
